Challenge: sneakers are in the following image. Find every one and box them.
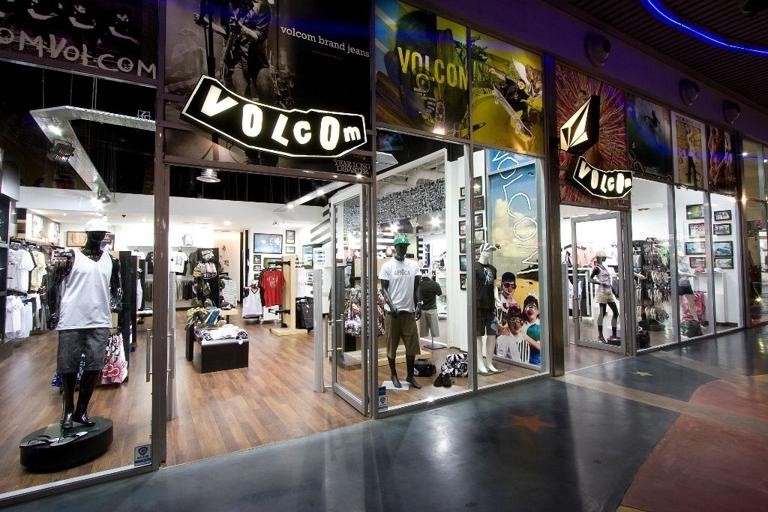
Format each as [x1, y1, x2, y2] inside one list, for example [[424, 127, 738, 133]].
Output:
[[434, 372, 451, 387]]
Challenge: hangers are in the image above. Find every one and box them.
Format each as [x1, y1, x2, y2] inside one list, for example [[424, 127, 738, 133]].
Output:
[[6, 290, 33, 305], [131, 247, 142, 252], [8, 238, 44, 252], [175, 247, 183, 253]]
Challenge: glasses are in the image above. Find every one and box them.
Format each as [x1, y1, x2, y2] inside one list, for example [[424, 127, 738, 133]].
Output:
[[504, 282, 516, 289]]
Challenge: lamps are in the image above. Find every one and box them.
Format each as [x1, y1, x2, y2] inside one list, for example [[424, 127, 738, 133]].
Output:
[[679, 77, 701, 109], [42, 137, 77, 164], [194, 167, 221, 184], [93, 181, 111, 205], [720, 99, 742, 127], [585, 30, 610, 70]]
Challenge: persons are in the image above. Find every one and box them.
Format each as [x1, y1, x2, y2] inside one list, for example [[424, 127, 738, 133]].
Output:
[[487, 63, 535, 130], [677, 251, 699, 323], [706, 127, 734, 191], [0, 2, 141, 63], [685, 123, 696, 187], [193, 0, 281, 168], [588, 250, 619, 345], [474, 242, 500, 376], [44, 217, 120, 432], [492, 272, 541, 366], [379, 236, 423, 390], [415, 272, 442, 339]]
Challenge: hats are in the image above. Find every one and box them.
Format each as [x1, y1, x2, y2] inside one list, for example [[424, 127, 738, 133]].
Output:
[[595, 250, 612, 259], [392, 236, 410, 245], [85, 219, 110, 233], [480, 244, 497, 253]]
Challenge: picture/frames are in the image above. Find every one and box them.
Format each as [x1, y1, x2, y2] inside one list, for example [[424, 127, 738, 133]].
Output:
[[456, 174, 492, 294], [65, 231, 89, 249], [682, 202, 738, 273], [250, 230, 297, 288]]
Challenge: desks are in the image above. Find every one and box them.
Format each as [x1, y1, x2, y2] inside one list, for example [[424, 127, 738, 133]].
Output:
[[191, 325, 249, 376]]
